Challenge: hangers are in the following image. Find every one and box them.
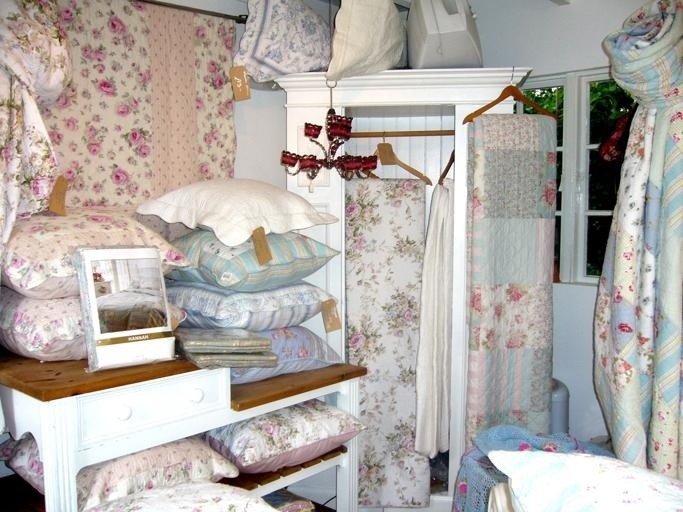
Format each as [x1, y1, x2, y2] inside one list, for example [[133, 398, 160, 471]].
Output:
[[353, 130, 431, 185], [458, 64, 561, 125], [438, 148, 459, 187]]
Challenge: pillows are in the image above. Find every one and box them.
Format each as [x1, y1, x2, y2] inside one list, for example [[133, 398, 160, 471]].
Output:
[[167, 286, 336, 333], [485, 446, 683, 512], [205, 397, 370, 475], [0, 285, 182, 363], [409, 1, 485, 70], [165, 231, 340, 293], [87, 480, 276, 512], [137, 176, 338, 248], [1, 204, 190, 300], [327, 0, 408, 86], [230, 328, 346, 386], [234, 0, 332, 82], [0, 436, 241, 511]]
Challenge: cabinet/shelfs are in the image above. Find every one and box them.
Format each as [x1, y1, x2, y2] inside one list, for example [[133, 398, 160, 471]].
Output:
[[0, 357, 370, 512], [275, 67, 532, 512]]
